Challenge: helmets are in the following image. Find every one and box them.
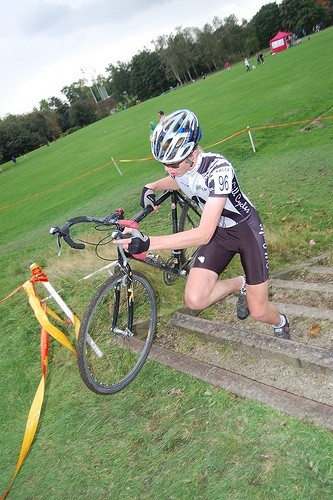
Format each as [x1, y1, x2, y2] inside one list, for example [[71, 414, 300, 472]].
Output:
[[150, 109, 202, 164]]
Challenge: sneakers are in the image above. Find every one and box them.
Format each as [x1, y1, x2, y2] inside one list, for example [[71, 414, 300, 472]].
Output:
[[273, 313, 289, 340], [236, 275, 250, 320]]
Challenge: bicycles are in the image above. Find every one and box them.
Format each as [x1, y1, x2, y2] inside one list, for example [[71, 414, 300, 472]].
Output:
[[49, 190, 203, 395]]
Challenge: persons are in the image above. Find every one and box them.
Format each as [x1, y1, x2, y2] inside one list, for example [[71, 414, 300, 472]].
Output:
[[149, 121, 156, 138], [224, 62, 232, 71], [314, 22, 325, 33], [113, 109, 290, 339], [200, 70, 206, 79], [287, 32, 299, 47], [244, 58, 250, 72], [256, 52, 265, 65], [303, 29, 307, 36], [157, 111, 166, 121]]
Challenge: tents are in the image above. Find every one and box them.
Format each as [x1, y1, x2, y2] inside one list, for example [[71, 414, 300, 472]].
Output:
[[269, 30, 292, 54]]
